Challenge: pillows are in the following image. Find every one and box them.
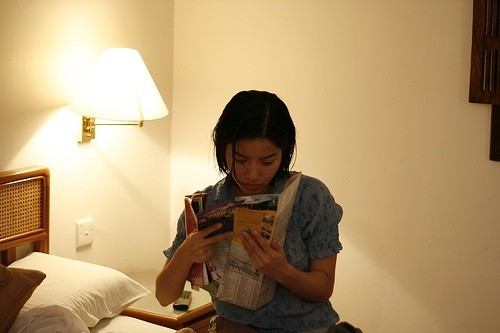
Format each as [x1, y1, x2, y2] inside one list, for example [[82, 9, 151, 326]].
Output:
[[6, 252, 152, 327], [0, 264, 46, 333]]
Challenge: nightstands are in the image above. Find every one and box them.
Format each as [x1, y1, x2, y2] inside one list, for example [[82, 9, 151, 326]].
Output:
[[120, 281, 218, 333]]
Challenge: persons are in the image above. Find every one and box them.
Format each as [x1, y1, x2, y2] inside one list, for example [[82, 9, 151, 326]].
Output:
[[156, 90, 363, 333]]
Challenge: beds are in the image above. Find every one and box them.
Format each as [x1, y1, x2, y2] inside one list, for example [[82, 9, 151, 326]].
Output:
[[0, 165, 177, 333]]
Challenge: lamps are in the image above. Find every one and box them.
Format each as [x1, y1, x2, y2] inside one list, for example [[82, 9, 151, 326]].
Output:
[[78, 48, 170, 144]]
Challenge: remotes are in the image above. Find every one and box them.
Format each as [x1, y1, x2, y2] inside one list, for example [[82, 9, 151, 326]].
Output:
[[173, 290, 192, 311]]
